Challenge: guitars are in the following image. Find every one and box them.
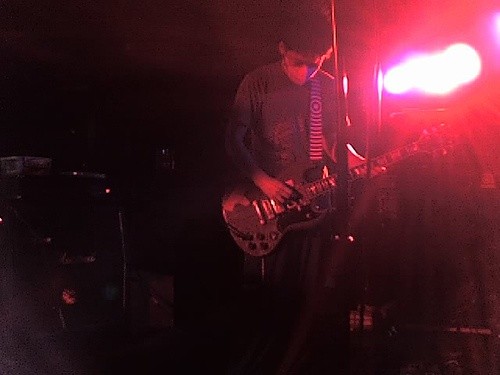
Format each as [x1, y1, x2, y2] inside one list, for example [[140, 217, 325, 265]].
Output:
[[222, 121, 457, 258]]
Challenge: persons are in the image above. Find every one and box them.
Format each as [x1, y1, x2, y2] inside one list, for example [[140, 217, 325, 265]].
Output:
[[221, 10, 387, 313]]
